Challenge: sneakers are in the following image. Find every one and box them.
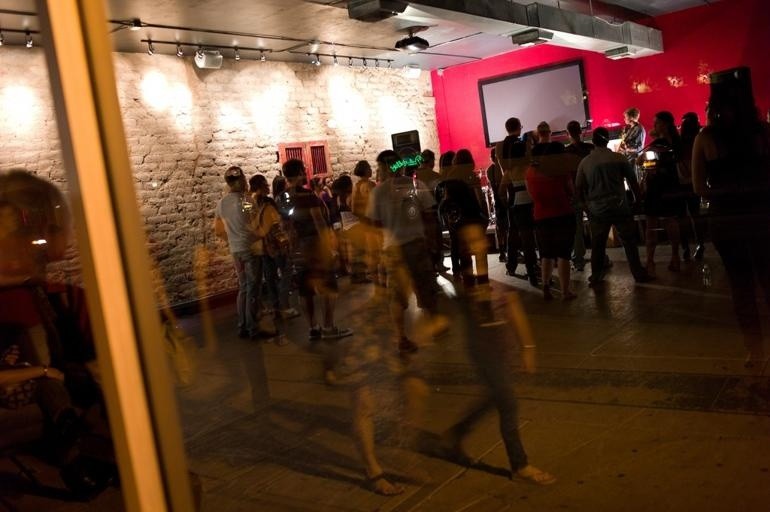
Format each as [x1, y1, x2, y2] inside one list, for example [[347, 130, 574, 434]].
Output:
[[398, 339, 418, 352], [308, 324, 353, 339], [438, 266, 450, 275], [499, 254, 584, 301], [636, 273, 656, 282], [238, 308, 300, 339]]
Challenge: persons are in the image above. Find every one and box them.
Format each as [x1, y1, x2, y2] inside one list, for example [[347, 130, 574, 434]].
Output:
[[417, 220, 557, 485], [0, 200, 46, 289], [691, 82, 770, 369], [211, 148, 489, 343], [486, 104, 701, 303], [308, 178, 429, 499], [0, 342, 76, 423], [147, 241, 217, 356]]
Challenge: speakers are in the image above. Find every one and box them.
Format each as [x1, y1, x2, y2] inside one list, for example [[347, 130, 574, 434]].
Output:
[[193, 48, 224, 70], [392, 130, 422, 160]]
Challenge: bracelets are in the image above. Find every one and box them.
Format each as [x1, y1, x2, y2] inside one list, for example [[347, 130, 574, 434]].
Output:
[[23, 272, 32, 288], [521, 343, 538, 349], [41, 364, 49, 377]]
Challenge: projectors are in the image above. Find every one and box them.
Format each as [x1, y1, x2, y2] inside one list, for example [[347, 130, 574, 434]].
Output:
[[394, 31, 431, 56]]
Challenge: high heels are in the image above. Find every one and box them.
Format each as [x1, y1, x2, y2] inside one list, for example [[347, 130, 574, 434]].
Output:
[[694, 243, 704, 258]]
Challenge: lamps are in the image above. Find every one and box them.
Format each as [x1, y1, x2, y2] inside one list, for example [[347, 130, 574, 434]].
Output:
[[0, 30, 5, 47], [140, 38, 273, 70], [24, 30, 33, 48], [289, 50, 395, 70]]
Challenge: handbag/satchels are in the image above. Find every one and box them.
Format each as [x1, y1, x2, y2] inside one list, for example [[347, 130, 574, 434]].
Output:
[[340, 212, 362, 234], [265, 224, 292, 258]]
